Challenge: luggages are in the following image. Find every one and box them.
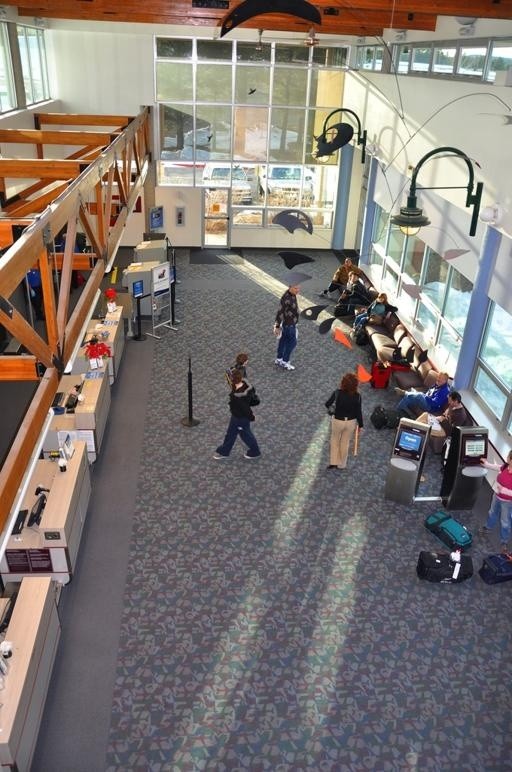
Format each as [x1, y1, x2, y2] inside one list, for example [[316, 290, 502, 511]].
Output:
[[371, 362, 391, 388]]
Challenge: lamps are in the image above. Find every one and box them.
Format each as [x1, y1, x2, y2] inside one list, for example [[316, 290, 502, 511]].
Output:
[[311, 108, 367, 165], [389, 147, 485, 237]]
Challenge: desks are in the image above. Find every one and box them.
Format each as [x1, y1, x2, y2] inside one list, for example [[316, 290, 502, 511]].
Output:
[[0, 286, 127, 772]]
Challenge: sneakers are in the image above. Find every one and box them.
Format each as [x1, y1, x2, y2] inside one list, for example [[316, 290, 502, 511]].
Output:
[[394, 387, 404, 396], [213, 453, 227, 460], [275, 358, 283, 365], [279, 361, 295, 370], [326, 291, 332, 299], [243, 452, 261, 459], [478, 526, 493, 533], [501, 544, 508, 551]]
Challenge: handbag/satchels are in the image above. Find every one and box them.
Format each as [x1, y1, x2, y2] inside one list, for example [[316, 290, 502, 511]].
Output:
[[357, 332, 369, 345], [370, 407, 387, 430], [478, 552, 512, 585], [328, 392, 337, 415], [416, 550, 474, 584], [368, 314, 382, 326], [334, 304, 348, 316]]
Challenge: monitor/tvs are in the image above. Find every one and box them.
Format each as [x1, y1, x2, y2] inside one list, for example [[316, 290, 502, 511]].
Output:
[[464, 439, 485, 458], [27, 494, 46, 527], [398, 431, 422, 454]]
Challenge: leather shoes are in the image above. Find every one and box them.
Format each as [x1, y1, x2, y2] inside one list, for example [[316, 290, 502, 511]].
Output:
[[327, 465, 336, 469]]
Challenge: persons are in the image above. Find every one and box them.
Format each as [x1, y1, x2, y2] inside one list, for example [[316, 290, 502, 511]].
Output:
[[350, 292, 399, 336], [392, 370, 449, 413], [226, 353, 256, 422], [338, 273, 371, 318], [212, 369, 262, 460], [324, 372, 364, 470], [479, 449, 512, 555], [338, 270, 354, 305], [274, 281, 301, 371], [317, 256, 363, 300], [413, 390, 467, 438]]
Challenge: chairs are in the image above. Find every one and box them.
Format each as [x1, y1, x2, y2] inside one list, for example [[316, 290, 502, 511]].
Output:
[[340, 268, 473, 455]]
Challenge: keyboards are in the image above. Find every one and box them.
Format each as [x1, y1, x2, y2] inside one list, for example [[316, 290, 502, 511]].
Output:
[[12, 510, 28, 534]]
